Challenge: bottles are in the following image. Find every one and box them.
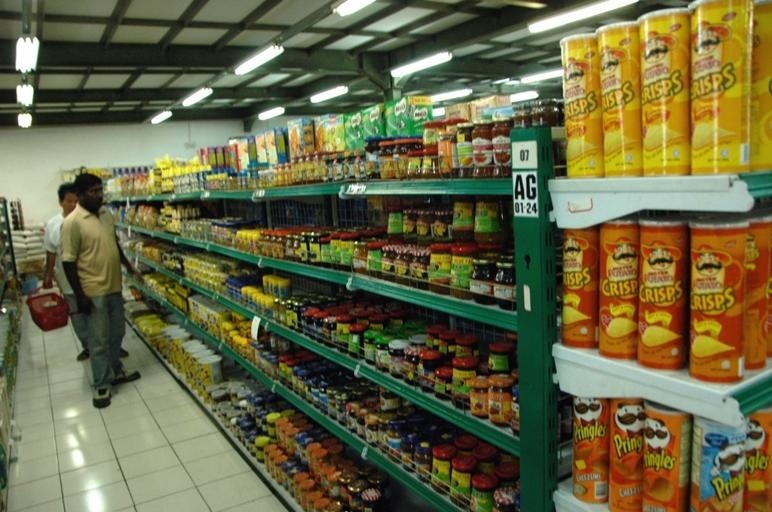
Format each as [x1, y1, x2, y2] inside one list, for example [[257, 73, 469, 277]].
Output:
[[111, 166, 152, 197], [356, 404, 521, 512], [228, 149, 368, 190], [364, 117, 512, 179], [222, 311, 290, 380], [272, 290, 520, 436], [511, 97, 565, 130], [205, 380, 384, 512], [206, 170, 228, 190]]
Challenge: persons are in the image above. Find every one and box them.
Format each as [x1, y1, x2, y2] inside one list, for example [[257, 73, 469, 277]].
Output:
[[43, 182, 128, 360], [62, 174, 141, 409]]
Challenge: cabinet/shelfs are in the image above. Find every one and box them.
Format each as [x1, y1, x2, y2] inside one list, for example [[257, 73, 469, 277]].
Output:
[[104, 127, 574, 511], [0, 196, 22, 313], [547, 171, 772, 512]]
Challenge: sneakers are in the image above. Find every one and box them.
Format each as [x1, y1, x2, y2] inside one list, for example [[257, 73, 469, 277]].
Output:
[[93, 386, 110, 408], [109, 370, 142, 385], [118, 348, 128, 357], [76, 350, 89, 361]]
